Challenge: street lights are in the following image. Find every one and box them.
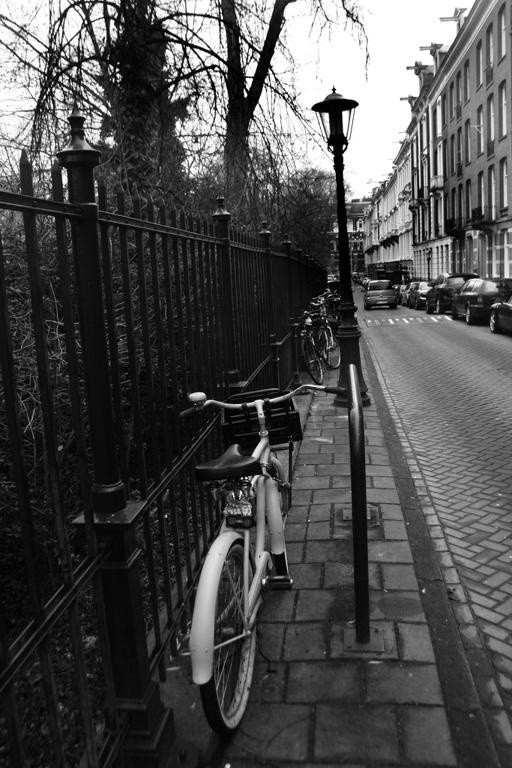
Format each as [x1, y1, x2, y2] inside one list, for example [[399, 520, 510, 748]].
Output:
[[310, 81, 372, 409]]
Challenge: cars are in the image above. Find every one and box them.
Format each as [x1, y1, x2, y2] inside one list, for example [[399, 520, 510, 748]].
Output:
[[351, 269, 512, 337]]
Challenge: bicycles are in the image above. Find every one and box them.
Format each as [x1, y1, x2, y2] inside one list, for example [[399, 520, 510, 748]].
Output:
[[295, 280, 340, 385], [178, 381, 348, 740]]
